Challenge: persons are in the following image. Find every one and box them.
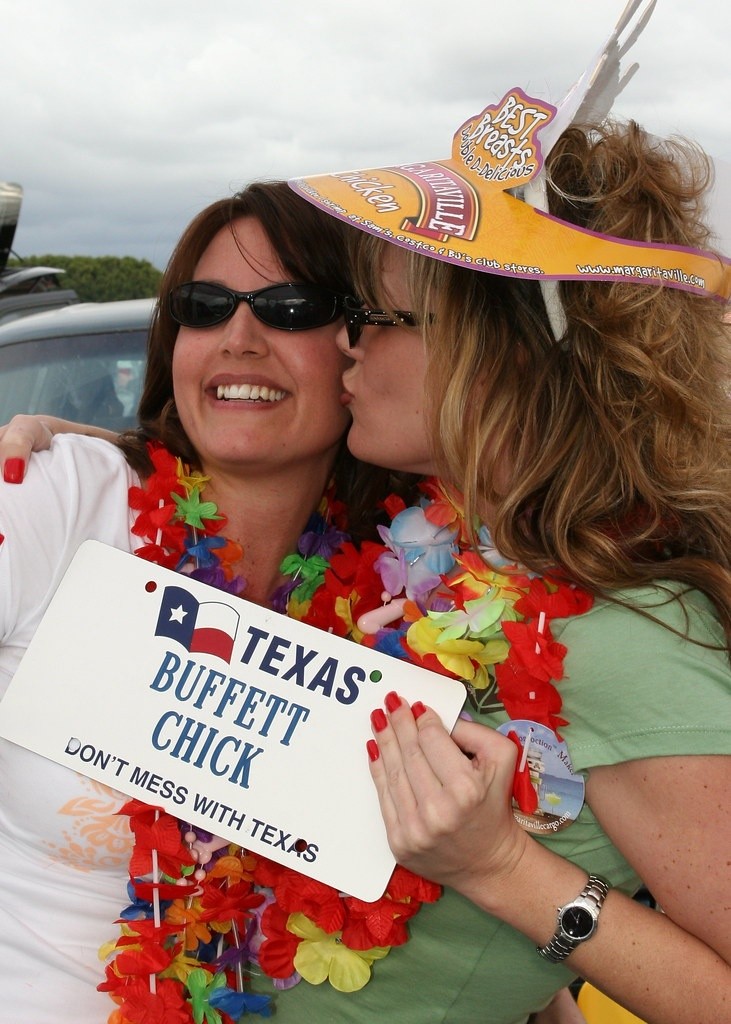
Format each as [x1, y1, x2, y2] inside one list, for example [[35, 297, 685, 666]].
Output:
[[0, 180, 425, 1023], [0, 118, 731, 1024]]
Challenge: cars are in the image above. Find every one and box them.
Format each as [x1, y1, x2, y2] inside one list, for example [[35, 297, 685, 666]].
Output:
[[0, 176, 166, 458]]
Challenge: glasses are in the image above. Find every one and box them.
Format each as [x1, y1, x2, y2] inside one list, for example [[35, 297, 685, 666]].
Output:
[[343, 296, 452, 350], [168, 279, 348, 332]]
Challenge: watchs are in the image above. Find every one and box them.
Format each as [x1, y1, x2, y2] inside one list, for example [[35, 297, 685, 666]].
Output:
[[537, 873, 609, 964]]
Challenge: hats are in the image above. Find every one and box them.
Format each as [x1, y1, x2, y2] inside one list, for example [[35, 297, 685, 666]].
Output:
[[283, 88, 731, 303]]
